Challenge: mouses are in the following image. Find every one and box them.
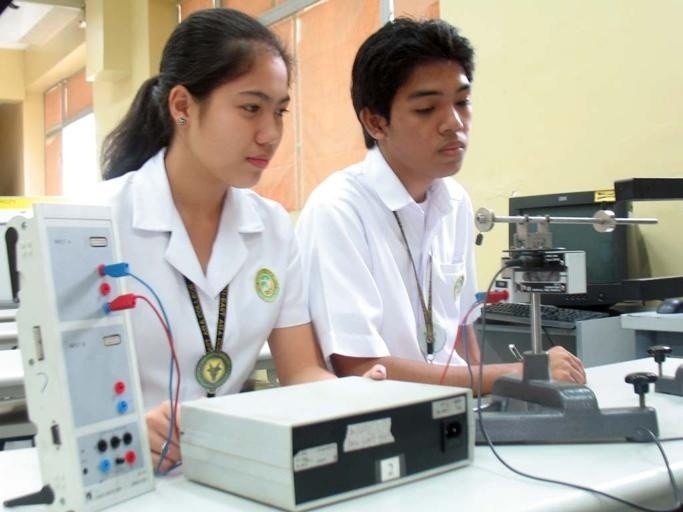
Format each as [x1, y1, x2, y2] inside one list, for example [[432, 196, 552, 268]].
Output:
[[656, 298, 683, 313]]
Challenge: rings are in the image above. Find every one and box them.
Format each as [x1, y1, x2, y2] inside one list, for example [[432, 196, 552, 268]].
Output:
[[160, 440, 173, 458]]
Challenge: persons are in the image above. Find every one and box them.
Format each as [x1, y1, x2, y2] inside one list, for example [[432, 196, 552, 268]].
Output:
[[299, 18, 587, 397], [56, 7, 388, 476]]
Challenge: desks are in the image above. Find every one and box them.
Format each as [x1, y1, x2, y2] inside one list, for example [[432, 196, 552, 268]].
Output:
[[2, 351, 680, 512]]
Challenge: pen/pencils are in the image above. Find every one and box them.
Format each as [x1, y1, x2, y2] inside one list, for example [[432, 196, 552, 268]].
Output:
[[509, 344, 525, 362]]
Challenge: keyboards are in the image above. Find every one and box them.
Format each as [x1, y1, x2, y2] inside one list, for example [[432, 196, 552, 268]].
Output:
[[481, 303, 610, 330]]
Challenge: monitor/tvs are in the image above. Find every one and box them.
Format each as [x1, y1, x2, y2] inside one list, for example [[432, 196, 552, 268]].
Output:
[[509, 190, 645, 307]]
